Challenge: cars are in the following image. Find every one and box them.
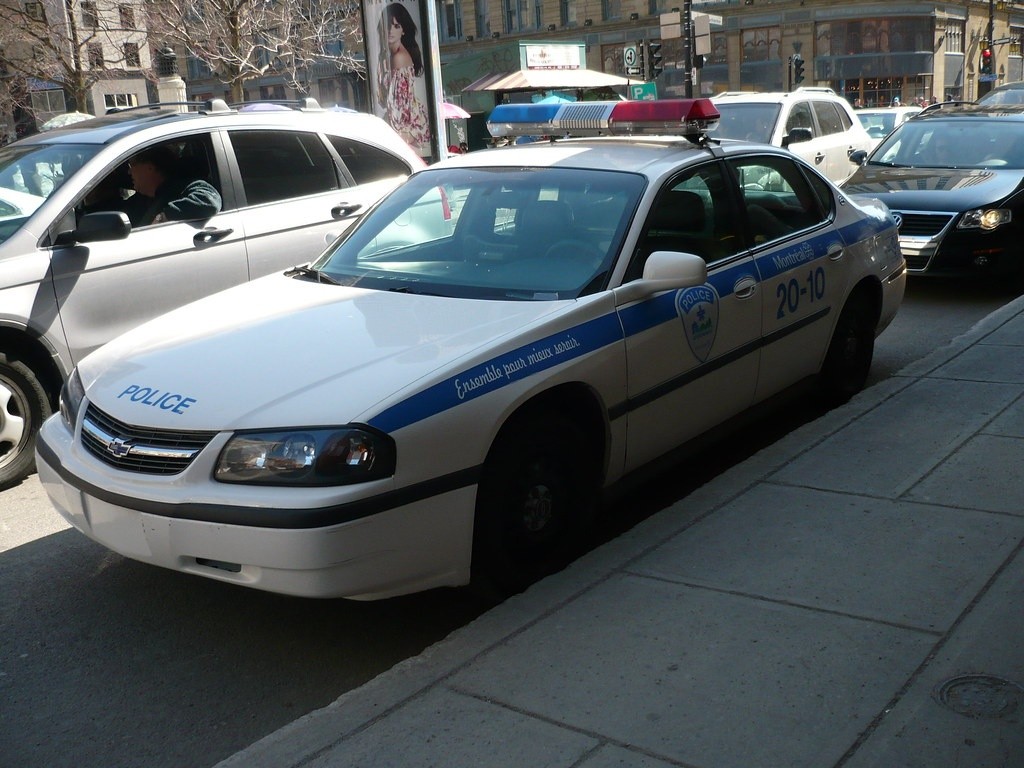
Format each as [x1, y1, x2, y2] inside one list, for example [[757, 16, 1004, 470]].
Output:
[[835, 100, 1024, 296], [31, 97, 907, 610], [851, 106, 937, 167], [969, 79, 1024, 110]]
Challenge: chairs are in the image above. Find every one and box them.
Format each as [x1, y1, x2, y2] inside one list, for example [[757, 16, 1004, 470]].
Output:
[[621, 188, 708, 281], [519, 198, 574, 254]]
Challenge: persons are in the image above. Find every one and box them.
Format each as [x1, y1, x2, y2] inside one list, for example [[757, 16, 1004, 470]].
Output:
[[919, 96, 927, 108], [929, 96, 941, 110], [851, 98, 863, 109], [119, 139, 222, 225], [376, 3, 430, 148], [890, 96, 907, 107], [926, 132, 958, 165], [910, 96, 923, 108], [945, 95, 955, 107]]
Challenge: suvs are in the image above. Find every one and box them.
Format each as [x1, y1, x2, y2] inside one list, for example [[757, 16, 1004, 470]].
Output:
[[671, 84, 878, 198], [0, 96, 456, 487]]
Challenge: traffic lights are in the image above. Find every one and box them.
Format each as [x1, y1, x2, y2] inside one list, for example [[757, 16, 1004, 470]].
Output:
[[795, 58, 804, 84], [647, 43, 662, 79], [981, 47, 993, 75]]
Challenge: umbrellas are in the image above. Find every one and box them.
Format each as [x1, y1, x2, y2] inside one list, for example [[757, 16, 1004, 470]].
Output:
[[41, 110, 96, 133], [443, 99, 471, 145], [237, 103, 293, 112]]
